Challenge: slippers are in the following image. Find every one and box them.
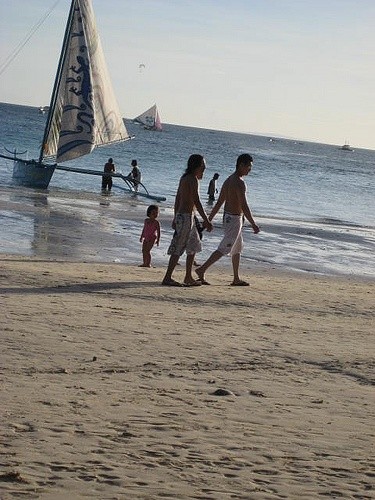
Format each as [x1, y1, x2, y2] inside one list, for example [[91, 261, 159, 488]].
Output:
[[230, 281, 249, 286], [196, 279, 211, 285]]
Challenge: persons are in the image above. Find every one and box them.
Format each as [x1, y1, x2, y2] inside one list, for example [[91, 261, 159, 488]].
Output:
[[128, 159, 142, 191], [195, 153, 260, 286], [176, 173, 220, 267], [102, 158, 116, 191], [138, 205, 161, 267], [161, 153, 212, 287]]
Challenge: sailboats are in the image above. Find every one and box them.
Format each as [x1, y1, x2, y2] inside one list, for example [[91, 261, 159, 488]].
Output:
[[10, 0, 131, 190], [133, 104, 164, 133]]
